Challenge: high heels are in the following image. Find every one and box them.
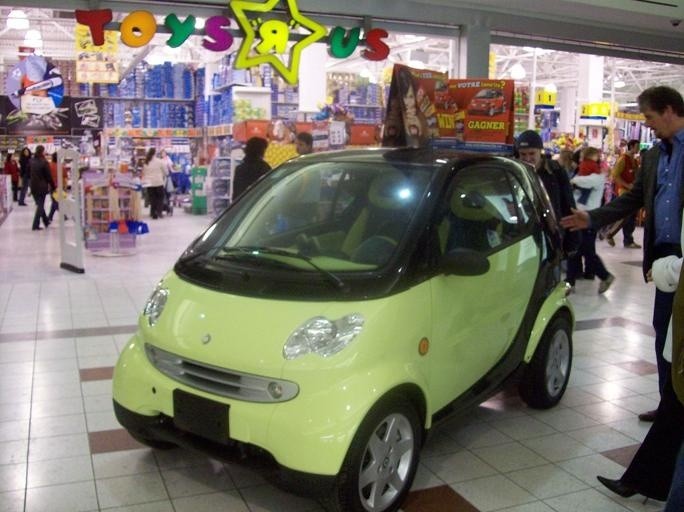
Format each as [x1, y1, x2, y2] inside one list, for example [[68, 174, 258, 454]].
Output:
[[597, 476, 650, 505]]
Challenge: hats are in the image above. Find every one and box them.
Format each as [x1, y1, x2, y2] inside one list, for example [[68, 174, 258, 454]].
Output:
[[515, 130, 542, 149]]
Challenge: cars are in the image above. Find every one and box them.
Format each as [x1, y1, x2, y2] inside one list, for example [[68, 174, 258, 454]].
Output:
[[433, 81, 449, 110], [468, 87, 506, 116], [110, 145, 577, 512]]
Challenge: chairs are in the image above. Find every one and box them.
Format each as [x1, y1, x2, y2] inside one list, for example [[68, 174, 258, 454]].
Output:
[[341, 166, 409, 273], [442, 182, 506, 257]]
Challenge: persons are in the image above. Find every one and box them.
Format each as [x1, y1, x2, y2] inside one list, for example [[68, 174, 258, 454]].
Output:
[[3, 145, 68, 231], [141, 148, 176, 220], [597, 207, 684, 505], [559, 86, 684, 422], [514, 129, 647, 294], [233, 136, 272, 201], [295, 132, 314, 154], [400, 70, 428, 142]]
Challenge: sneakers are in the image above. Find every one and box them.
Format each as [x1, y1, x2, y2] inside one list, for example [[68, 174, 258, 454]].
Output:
[[599, 274, 614, 294], [624, 243, 641, 248], [602, 232, 615, 246], [583, 272, 595, 279], [639, 411, 657, 422]]
